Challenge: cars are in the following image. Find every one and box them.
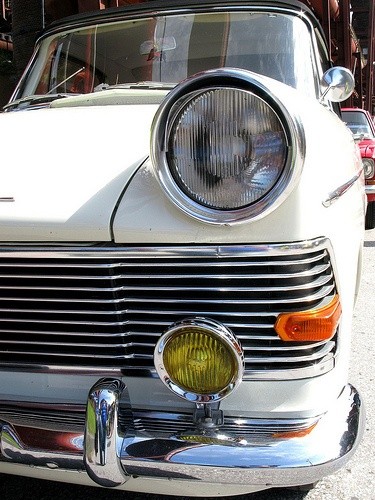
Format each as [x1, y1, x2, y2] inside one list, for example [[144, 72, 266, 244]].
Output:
[[0, 0, 373, 500], [337, 106, 373, 200]]
